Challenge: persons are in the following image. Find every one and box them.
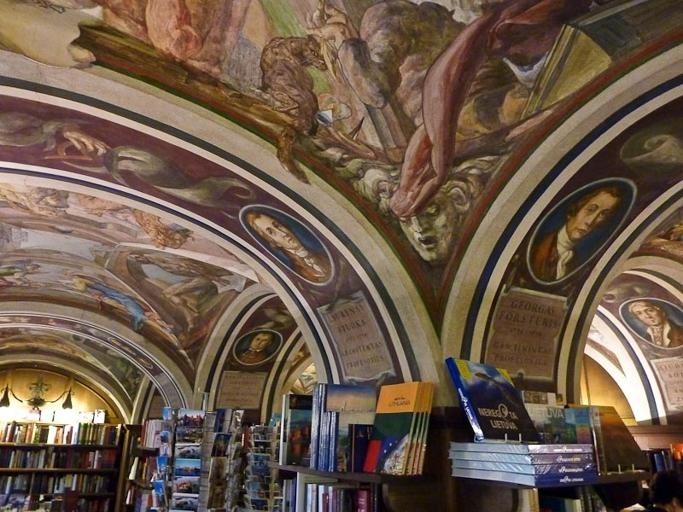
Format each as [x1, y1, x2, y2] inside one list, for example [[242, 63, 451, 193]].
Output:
[[532, 183, 624, 281], [236, 332, 275, 362], [397, 190, 459, 267], [629, 301, 683, 348], [245, 210, 331, 283]]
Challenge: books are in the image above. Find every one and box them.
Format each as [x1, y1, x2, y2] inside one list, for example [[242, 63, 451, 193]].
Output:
[[445, 357, 649, 486], [0, 420, 121, 512], [279, 381, 436, 512], [126, 407, 283, 512]]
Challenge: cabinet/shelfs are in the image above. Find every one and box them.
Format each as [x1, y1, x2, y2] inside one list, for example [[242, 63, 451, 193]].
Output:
[[119, 444, 157, 511], [0, 424, 125, 512], [265, 459, 649, 512]]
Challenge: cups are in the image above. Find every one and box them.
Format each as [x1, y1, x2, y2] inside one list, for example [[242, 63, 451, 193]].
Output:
[[661, 450, 675, 473], [654, 453, 665, 472]]
